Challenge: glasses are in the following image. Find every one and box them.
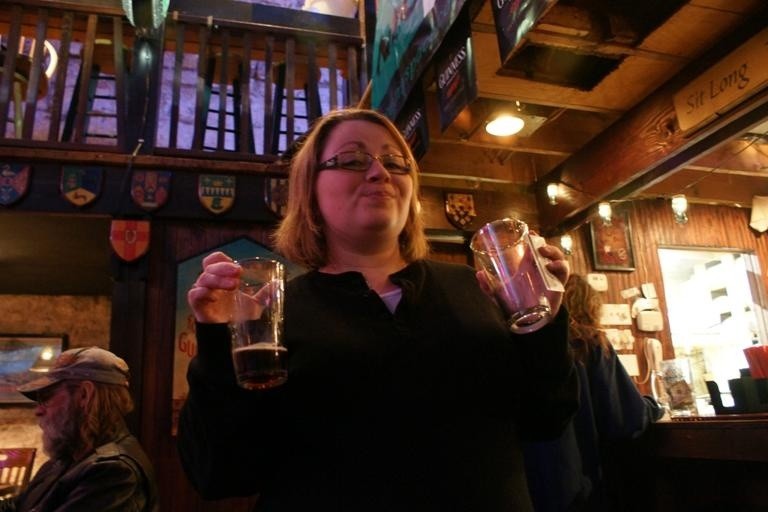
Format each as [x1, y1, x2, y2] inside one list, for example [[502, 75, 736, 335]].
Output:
[[314, 150, 408, 173]]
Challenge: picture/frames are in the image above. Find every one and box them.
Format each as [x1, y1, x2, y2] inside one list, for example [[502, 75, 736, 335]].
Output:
[[590, 209, 636, 272], [0, 333, 69, 408]]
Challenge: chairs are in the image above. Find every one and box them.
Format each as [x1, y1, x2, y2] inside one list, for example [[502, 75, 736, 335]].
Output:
[[0, 448, 38, 501]]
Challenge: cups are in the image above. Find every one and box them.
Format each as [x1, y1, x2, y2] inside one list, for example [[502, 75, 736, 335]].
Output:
[[230, 257, 287, 390], [470, 218, 554, 332]]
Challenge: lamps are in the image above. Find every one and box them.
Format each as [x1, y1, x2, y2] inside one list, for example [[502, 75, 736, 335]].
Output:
[[672, 192, 688, 225]]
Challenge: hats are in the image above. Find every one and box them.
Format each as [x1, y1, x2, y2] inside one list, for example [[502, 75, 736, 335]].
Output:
[[15, 346, 130, 401]]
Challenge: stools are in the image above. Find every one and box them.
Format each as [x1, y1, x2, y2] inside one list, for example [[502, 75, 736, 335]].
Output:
[[2, 52, 48, 139], [61, 39, 322, 155]]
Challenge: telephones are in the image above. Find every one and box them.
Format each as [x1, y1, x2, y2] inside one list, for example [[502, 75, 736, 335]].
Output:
[[644, 338, 662, 373]]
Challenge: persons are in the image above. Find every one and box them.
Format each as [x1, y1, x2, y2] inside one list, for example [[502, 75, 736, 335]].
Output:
[[0, 345, 160, 512], [526, 273, 659, 512], [176, 108, 583, 512]]
